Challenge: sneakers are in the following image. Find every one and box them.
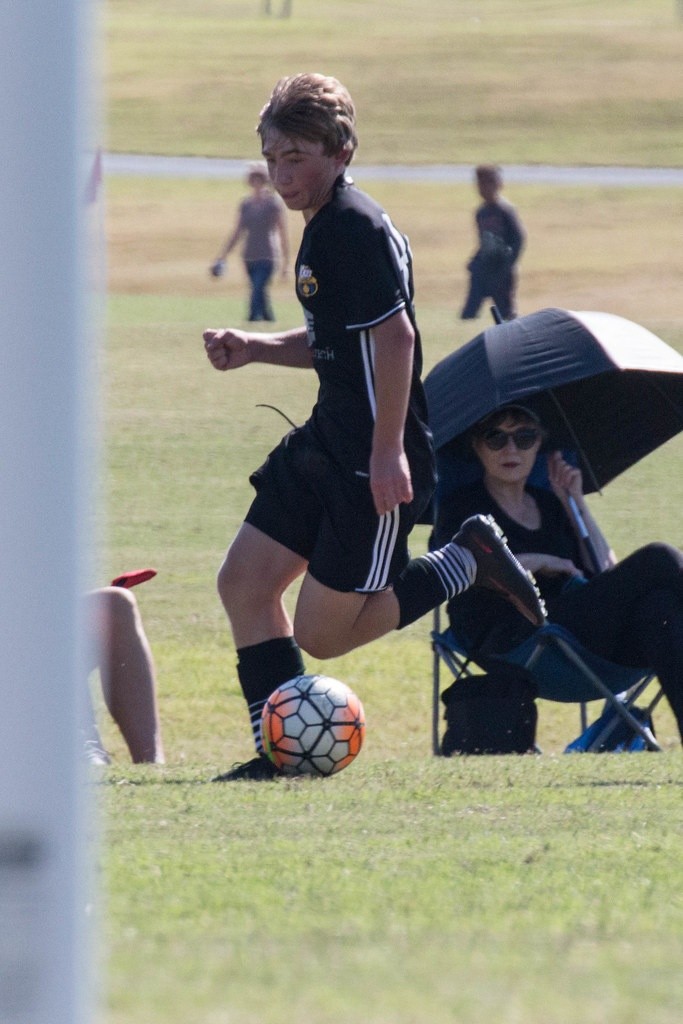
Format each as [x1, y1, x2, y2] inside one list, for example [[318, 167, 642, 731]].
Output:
[[452, 513, 547, 625], [213, 756, 278, 781]]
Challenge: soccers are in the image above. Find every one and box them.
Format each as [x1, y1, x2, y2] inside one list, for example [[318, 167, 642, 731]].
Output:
[[260, 675, 365, 777]]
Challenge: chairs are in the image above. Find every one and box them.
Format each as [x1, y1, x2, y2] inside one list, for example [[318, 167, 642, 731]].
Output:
[[430, 469, 669, 755]]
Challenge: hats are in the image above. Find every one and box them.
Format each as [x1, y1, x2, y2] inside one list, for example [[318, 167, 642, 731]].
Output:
[[477, 400, 542, 424]]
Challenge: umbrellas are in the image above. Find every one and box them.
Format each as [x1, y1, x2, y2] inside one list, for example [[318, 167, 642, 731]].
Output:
[[382, 302, 683, 535]]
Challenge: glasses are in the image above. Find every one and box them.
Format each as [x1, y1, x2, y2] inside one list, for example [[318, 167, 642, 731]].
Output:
[[479, 428, 540, 450]]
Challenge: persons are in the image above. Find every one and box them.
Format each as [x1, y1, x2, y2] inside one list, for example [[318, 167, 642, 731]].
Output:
[[435, 392, 683, 775], [208, 165, 298, 332], [454, 154, 536, 330], [79, 588, 171, 772], [197, 67, 551, 791]]
